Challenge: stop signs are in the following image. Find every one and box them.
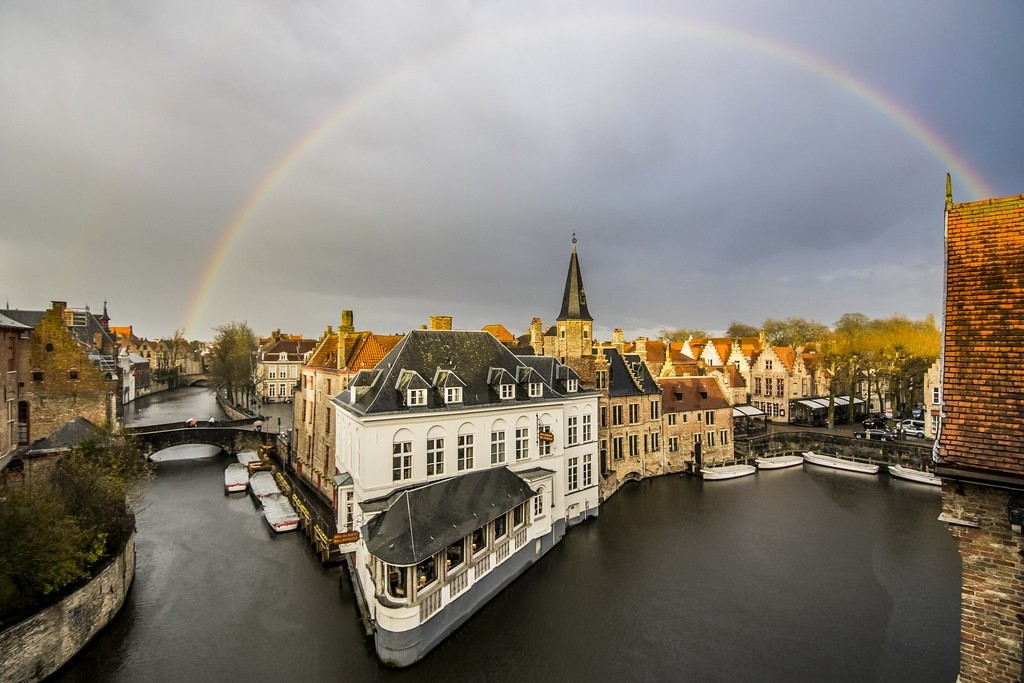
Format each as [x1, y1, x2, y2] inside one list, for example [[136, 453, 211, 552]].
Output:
[[885, 408, 892, 413]]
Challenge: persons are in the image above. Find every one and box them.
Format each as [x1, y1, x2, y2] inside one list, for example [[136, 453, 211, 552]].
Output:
[[208, 416, 215, 427]]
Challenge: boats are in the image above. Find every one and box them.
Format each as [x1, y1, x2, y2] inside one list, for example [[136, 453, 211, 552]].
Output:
[[802, 451, 880, 474], [754, 455, 804, 470], [699, 465, 756, 481], [887, 464, 942, 486], [236, 447, 263, 468], [224, 463, 250, 495], [248, 470, 301, 533]]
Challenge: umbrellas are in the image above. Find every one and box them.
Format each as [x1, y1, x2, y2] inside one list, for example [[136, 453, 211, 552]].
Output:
[[186, 418, 197, 426], [254, 420, 262, 427]]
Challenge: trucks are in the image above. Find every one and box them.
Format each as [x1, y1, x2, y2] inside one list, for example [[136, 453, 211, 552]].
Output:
[[911, 408, 924, 420]]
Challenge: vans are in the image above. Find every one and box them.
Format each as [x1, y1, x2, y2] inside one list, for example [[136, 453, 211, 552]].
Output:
[[896, 419, 925, 430]]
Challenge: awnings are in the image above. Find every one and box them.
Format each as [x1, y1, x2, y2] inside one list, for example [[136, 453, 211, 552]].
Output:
[[797, 395, 866, 411], [733, 404, 768, 420]]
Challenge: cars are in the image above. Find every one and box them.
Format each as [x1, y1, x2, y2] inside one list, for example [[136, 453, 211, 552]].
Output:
[[862, 416, 892, 428], [900, 425, 925, 438], [853, 428, 893, 442]]
